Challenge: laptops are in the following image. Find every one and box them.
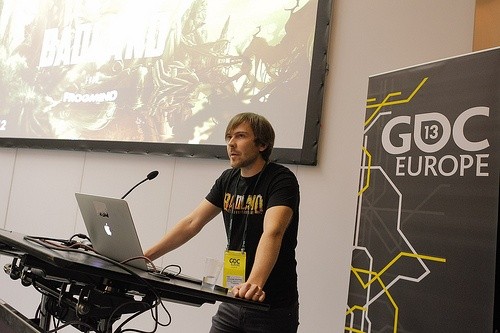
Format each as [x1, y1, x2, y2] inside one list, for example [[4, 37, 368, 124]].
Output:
[[75, 193, 203, 285]]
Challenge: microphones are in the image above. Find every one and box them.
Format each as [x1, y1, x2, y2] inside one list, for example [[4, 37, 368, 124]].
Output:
[[121, 171, 159, 199]]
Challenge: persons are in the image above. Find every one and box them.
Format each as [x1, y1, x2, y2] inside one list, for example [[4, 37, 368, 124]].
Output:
[[144, 113, 299, 333]]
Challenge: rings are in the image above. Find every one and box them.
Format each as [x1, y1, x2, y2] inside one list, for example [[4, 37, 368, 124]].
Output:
[[254, 293, 261, 296]]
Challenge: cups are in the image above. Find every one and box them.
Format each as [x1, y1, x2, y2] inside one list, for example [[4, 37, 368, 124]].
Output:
[[200, 257, 224, 293]]
